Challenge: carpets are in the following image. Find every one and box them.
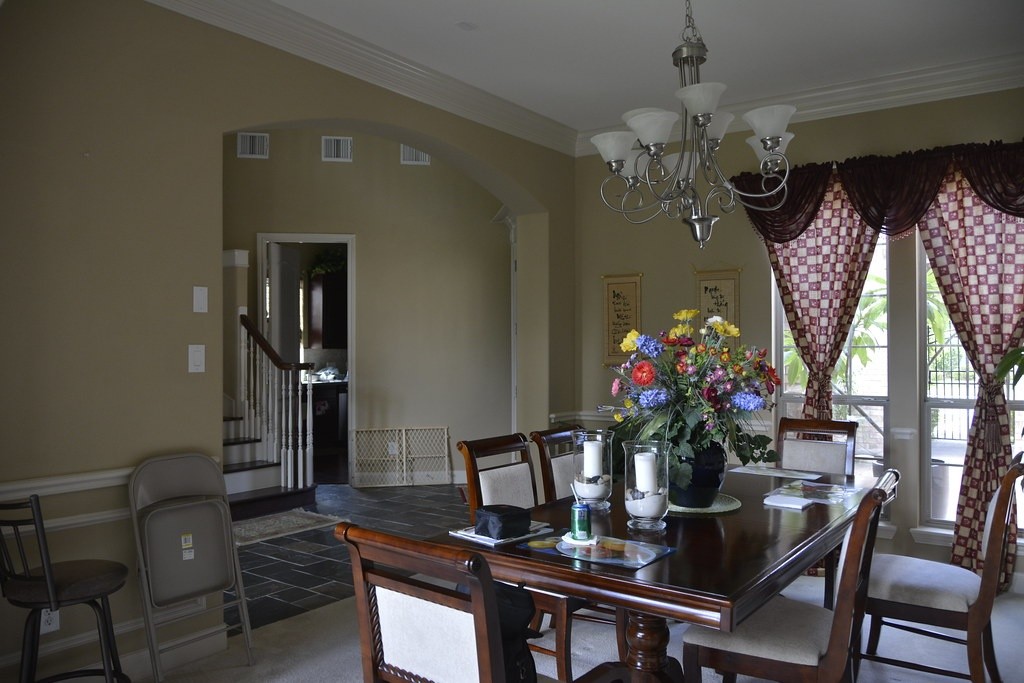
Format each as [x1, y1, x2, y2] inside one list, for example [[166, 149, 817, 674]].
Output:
[[232, 507, 351, 546]]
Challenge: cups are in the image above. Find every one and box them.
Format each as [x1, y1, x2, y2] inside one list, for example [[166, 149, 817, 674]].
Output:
[[301, 369, 309, 381]]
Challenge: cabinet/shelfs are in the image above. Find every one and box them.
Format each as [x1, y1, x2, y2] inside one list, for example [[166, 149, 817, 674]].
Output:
[[308, 271, 347, 350]]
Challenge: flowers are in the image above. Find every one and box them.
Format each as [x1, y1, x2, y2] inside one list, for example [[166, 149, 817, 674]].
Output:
[[595, 308, 782, 491]]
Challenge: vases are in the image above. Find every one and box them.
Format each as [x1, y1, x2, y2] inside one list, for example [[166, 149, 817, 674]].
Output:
[[668, 420, 728, 508]]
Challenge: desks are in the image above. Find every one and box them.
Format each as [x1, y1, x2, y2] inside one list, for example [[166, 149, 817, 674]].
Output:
[[423, 464, 898, 683]]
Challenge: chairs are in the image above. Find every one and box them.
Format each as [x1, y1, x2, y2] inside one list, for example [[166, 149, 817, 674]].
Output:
[[776, 417, 859, 611], [333, 521, 561, 683], [681, 468, 901, 683], [852, 452, 1024, 683], [456, 433, 590, 683], [530, 424, 684, 663], [128, 452, 257, 683], [0, 494, 131, 683]]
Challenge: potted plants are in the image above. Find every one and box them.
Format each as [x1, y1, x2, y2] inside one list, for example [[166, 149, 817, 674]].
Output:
[[783, 266, 951, 520]]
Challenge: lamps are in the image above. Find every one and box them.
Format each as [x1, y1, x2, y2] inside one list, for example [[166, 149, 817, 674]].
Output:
[[589, 0, 797, 251]]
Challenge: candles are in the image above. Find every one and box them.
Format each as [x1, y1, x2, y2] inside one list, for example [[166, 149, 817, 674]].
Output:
[[570, 429, 615, 511], [622, 439, 672, 531]]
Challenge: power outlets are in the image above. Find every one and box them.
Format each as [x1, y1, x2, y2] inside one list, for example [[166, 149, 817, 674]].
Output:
[[40, 608, 60, 635], [388, 442, 398, 455]]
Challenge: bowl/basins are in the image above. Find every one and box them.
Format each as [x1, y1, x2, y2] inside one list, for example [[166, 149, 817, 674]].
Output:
[[305, 373, 321, 382], [321, 373, 334, 381]]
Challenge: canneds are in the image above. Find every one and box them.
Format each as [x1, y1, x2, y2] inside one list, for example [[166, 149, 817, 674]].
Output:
[[570, 503, 591, 540]]
